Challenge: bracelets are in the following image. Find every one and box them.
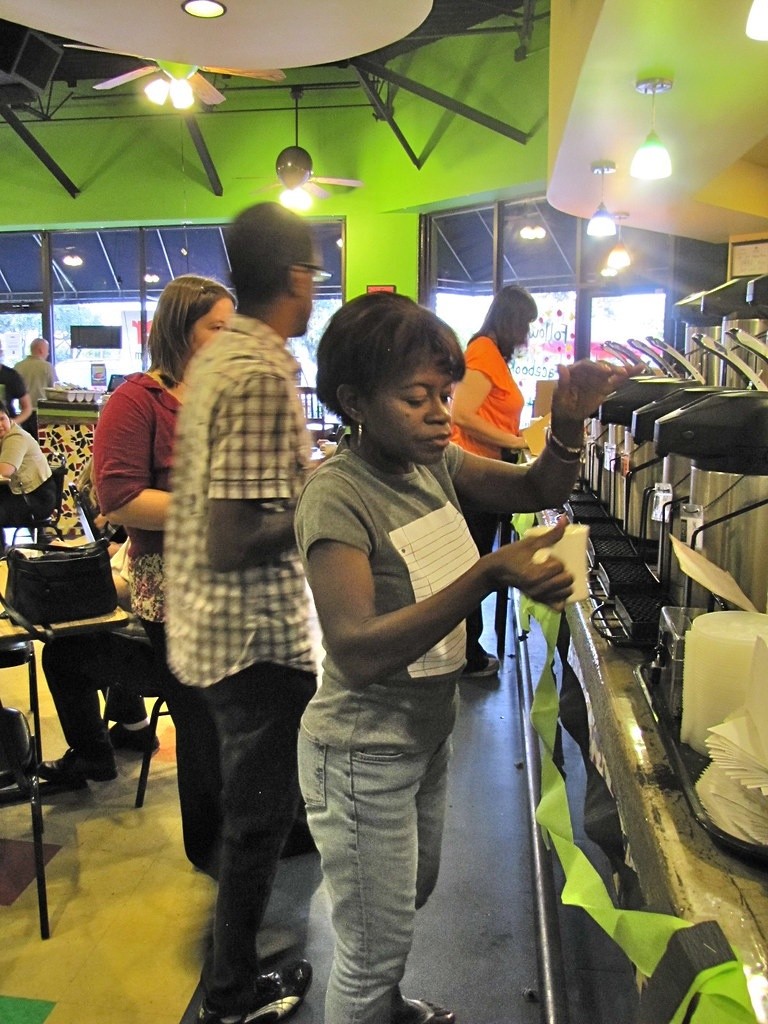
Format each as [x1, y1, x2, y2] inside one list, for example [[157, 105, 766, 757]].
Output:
[[545, 427, 586, 464]]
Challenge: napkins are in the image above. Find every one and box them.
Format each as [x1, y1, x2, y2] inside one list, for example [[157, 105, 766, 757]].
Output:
[[693, 634, 768, 848]]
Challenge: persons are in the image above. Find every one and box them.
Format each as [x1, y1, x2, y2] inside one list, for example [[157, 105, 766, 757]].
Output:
[[0, 364, 32, 426], [293, 291, 646, 1024], [0, 402, 56, 558], [446, 284, 538, 677], [36, 458, 163, 783], [12, 339, 60, 442], [165, 202, 321, 1024], [93, 275, 316, 876]]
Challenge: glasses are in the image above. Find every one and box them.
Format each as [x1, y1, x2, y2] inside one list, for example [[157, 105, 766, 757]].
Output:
[[289, 261, 332, 283]]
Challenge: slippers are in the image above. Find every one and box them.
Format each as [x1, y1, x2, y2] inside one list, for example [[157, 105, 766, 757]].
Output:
[[390, 999, 455, 1024]]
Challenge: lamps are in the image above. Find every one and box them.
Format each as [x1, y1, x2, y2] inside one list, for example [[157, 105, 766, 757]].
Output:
[[586, 159, 617, 238], [144, 76, 195, 108], [627, 77, 673, 181], [607, 211, 631, 270], [279, 186, 311, 211], [519, 224, 546, 239]]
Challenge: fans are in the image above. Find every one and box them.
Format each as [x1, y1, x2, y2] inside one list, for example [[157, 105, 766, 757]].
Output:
[[232, 89, 366, 199], [62, 43, 288, 105], [504, 210, 539, 223]]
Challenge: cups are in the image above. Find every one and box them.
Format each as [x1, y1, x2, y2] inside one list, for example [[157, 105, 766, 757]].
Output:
[[320, 442, 338, 455], [523, 523, 591, 606]]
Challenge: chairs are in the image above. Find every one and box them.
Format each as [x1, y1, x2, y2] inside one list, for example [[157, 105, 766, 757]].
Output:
[[12, 465, 65, 546], [103, 677, 172, 808], [0, 703, 52, 941], [0, 639, 43, 764]]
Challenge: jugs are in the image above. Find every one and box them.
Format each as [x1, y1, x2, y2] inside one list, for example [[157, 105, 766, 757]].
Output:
[[585, 323, 767, 615]]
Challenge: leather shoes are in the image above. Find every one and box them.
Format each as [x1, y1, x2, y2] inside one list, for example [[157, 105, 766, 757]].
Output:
[[108, 722, 159, 752], [37, 747, 118, 782]]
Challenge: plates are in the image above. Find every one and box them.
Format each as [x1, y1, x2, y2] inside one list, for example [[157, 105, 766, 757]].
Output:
[[680, 610, 768, 758]]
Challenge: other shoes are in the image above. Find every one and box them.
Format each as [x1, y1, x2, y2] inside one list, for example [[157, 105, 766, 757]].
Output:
[[461, 657, 500, 677], [484, 652, 496, 659]]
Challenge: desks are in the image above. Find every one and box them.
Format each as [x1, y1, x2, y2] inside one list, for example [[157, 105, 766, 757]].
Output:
[[1, 559, 130, 808]]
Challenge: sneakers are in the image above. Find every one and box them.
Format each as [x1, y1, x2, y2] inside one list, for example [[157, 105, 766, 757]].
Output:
[[197, 958, 313, 1024]]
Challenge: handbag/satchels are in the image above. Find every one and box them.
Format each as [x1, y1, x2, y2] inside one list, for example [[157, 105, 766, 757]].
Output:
[[4, 548, 118, 625]]
